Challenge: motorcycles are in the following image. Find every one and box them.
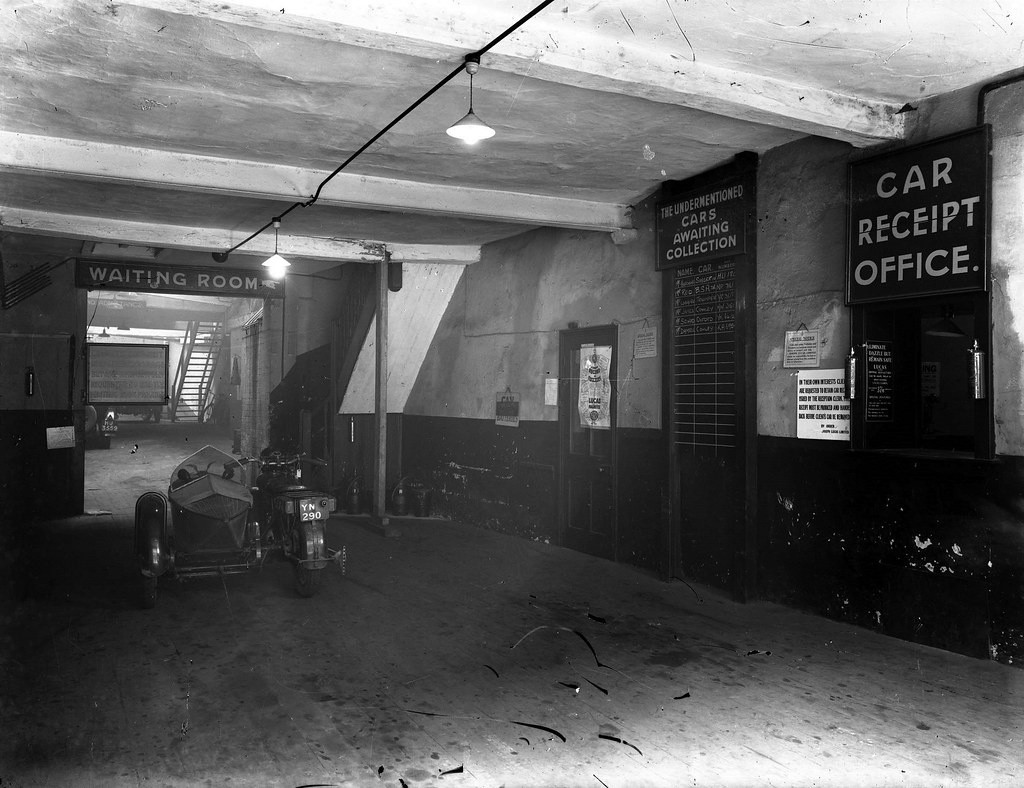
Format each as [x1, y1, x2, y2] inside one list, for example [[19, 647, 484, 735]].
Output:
[[86, 404, 117, 449], [133, 444, 336, 602]]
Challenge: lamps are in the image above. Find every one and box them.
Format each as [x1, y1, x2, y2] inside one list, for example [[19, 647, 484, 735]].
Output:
[[261, 218, 291, 280], [446, 55, 496, 146]]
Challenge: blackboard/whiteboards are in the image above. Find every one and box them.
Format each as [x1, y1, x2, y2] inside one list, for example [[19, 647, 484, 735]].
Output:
[[661, 255, 757, 457]]
